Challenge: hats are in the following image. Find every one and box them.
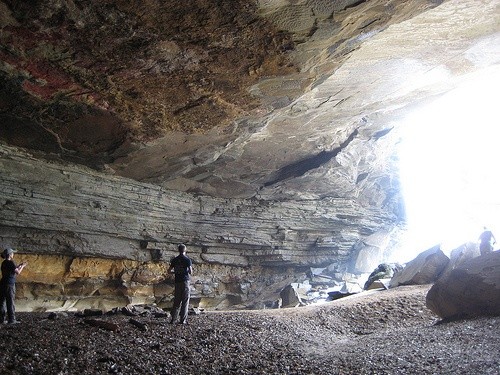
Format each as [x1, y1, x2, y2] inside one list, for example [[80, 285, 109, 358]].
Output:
[[4, 248, 18, 255]]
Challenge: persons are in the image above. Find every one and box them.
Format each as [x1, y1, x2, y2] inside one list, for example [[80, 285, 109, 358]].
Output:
[[1, 248, 28, 324], [167, 242, 194, 324]]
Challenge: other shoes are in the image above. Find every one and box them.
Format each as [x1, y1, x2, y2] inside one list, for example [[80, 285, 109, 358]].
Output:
[[9, 321, 21, 324], [170, 320, 188, 324], [0, 321, 8, 324]]
